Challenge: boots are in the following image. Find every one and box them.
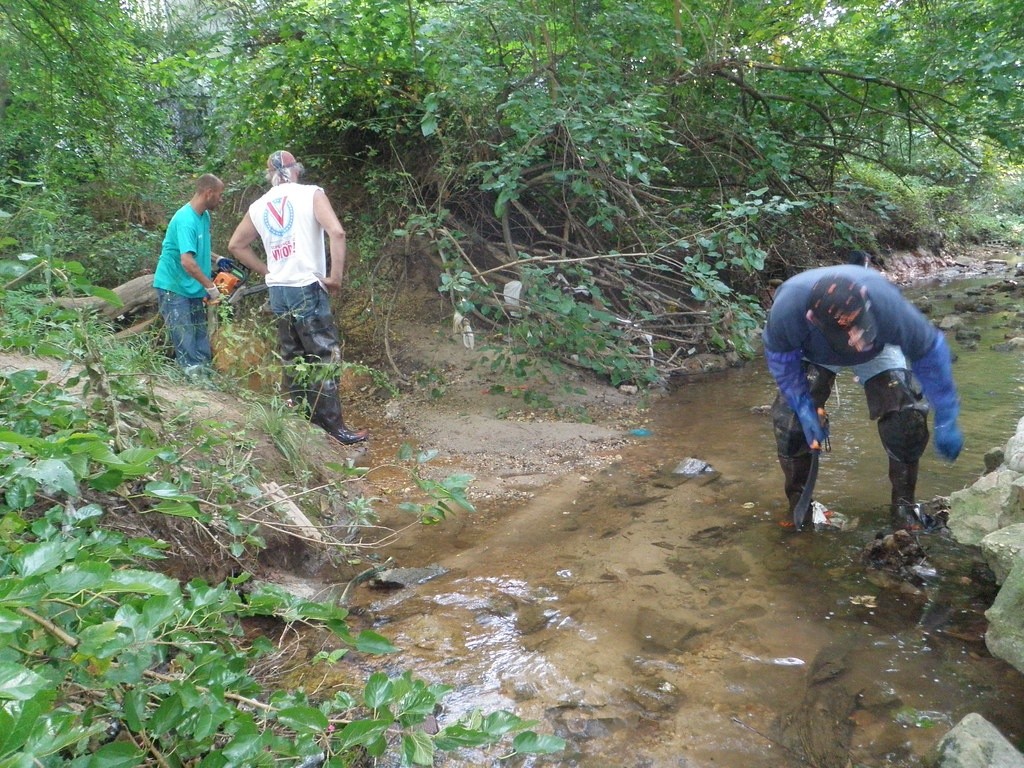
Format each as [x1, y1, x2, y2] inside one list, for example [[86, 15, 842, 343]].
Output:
[[779, 457, 815, 525], [280, 385, 310, 420], [306, 386, 369, 445], [889, 457, 926, 531]]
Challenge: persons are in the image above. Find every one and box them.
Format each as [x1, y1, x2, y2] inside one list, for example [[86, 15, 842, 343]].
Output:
[[227, 150, 370, 445], [761, 264, 964, 534], [153, 173, 235, 380]]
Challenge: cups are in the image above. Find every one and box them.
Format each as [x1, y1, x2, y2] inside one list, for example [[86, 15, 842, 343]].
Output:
[[216, 255, 247, 283]]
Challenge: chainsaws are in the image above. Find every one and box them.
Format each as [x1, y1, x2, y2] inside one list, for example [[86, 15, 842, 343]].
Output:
[[202, 262, 269, 306]]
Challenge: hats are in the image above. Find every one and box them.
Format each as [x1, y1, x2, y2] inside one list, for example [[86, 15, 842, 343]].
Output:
[[809, 274, 877, 356]]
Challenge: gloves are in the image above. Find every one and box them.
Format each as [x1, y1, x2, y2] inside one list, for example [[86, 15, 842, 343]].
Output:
[[216, 256, 235, 273], [205, 283, 220, 299], [910, 329, 964, 463], [765, 348, 830, 455]]
